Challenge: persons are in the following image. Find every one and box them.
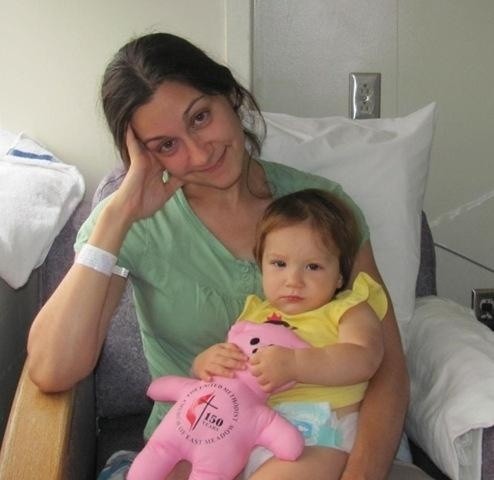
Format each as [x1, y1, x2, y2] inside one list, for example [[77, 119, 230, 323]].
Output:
[[159, 186, 392, 480], [24, 25, 435, 480]]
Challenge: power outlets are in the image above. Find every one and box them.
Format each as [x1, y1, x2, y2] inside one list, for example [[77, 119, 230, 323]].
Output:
[[346, 72, 382, 121]]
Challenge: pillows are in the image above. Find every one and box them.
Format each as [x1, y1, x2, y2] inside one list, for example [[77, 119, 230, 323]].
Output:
[[239, 98, 440, 466]]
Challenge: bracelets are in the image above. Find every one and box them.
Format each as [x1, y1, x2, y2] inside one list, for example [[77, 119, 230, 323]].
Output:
[[74, 243, 128, 278]]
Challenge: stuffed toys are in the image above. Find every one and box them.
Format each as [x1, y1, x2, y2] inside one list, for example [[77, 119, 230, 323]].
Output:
[[124, 319, 317, 480]]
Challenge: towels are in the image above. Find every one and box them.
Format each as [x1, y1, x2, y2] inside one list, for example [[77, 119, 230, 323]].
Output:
[[0, 128, 87, 291]]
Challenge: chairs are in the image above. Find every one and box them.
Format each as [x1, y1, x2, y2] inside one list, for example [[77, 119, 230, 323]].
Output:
[[1, 153, 493, 479]]
